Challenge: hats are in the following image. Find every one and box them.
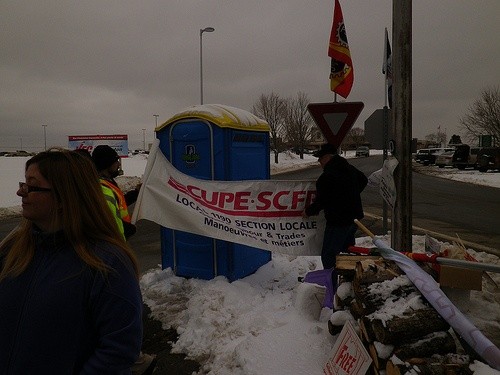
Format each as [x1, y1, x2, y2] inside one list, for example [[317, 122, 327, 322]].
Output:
[[312, 143, 337, 157], [92, 145, 120, 167]]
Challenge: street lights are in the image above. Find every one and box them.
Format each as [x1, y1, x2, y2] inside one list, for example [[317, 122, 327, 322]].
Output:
[[153, 114, 160, 128], [199, 27, 215, 105], [42, 124, 47, 151]]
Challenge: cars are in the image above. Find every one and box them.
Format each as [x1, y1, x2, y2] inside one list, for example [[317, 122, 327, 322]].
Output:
[[435, 150, 487, 169], [132, 149, 149, 155], [356, 147, 369, 157]]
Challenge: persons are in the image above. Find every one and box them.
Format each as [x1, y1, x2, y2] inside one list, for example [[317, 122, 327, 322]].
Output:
[[301, 145, 368, 294], [0, 142, 144, 375]]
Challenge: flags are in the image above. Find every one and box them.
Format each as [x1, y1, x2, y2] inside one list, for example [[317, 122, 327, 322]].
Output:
[[382, 32, 392, 109], [328, 0, 355, 99]]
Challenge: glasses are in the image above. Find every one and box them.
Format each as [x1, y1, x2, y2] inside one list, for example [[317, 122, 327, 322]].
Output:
[[19, 182, 52, 193]]
